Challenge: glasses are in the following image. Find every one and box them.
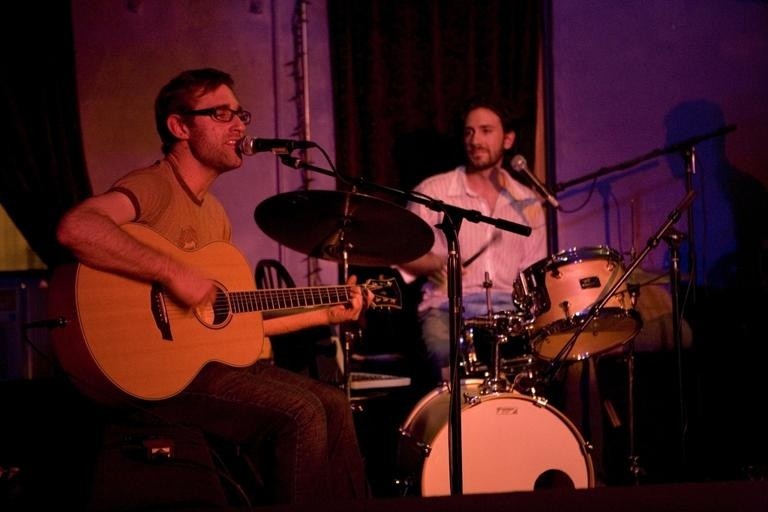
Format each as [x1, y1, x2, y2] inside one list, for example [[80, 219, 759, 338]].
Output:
[[189, 106, 251, 124]]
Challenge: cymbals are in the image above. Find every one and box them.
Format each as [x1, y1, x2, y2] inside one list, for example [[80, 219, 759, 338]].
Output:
[[254, 190, 433, 267]]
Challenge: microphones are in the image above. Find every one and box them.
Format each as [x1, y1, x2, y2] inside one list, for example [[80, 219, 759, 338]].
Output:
[[240, 134, 315, 156], [511, 155, 560, 208]]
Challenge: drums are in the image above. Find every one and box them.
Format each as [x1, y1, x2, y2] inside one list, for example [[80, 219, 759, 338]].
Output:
[[395, 378, 596, 498], [461, 312, 532, 375], [511, 247, 644, 364]]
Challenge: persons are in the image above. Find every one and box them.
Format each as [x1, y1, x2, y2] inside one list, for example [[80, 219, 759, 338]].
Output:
[[49, 65, 382, 508], [402, 99, 548, 369]]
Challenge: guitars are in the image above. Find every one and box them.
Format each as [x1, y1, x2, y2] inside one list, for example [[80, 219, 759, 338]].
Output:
[[49, 224, 401, 402]]
